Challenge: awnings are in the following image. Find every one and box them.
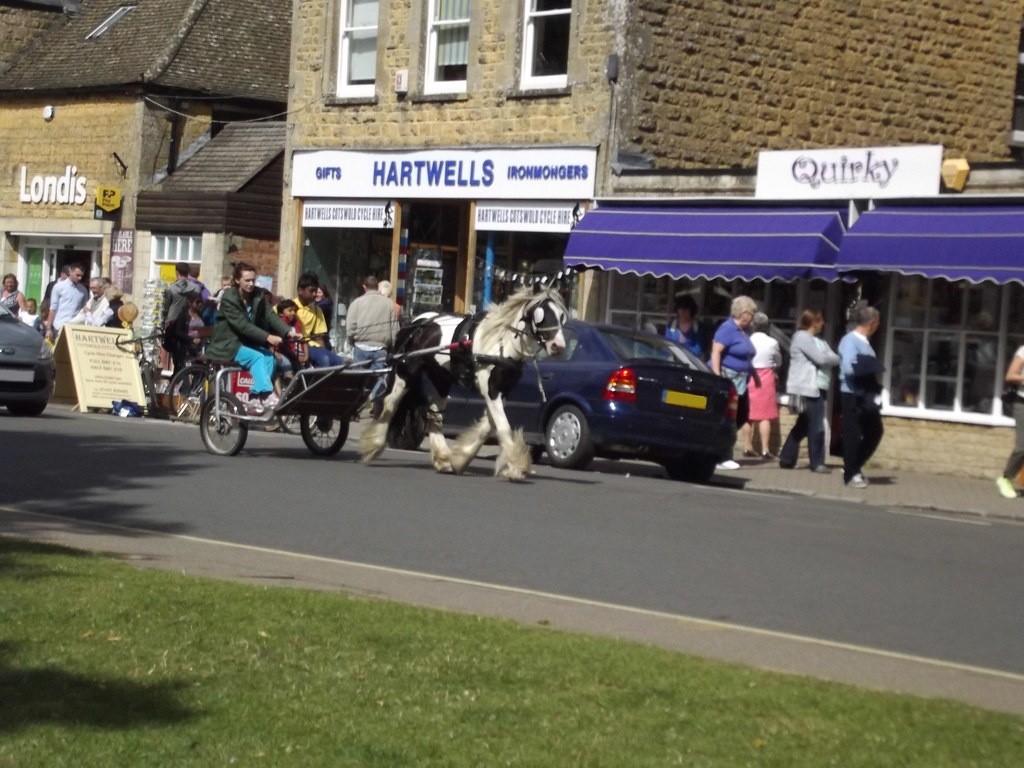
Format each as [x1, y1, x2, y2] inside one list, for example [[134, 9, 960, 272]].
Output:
[[561, 205, 847, 284], [834, 203, 1023, 289]]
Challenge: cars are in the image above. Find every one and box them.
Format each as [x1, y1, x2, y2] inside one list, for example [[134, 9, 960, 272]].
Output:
[[386, 318, 738, 486], [0, 298, 58, 417]]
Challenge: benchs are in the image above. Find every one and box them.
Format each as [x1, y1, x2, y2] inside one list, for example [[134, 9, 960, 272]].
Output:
[[196, 326, 254, 404]]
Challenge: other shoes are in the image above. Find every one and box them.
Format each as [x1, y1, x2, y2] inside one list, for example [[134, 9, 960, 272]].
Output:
[[814, 465, 832, 473], [846, 472, 869, 489], [996, 475, 1017, 499], [743, 450, 760, 458], [262, 392, 281, 409], [715, 459, 741, 470], [761, 452, 779, 461], [247, 398, 264, 414]]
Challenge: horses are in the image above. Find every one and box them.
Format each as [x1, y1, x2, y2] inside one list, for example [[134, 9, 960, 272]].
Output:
[[352, 275, 572, 484]]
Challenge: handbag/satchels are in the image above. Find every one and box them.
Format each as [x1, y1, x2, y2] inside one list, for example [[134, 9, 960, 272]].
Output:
[[1000, 382, 1019, 403], [44, 336, 54, 350]]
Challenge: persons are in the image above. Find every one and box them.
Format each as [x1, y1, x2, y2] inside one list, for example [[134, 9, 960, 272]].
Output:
[[204, 258, 294, 414], [778, 306, 840, 473], [837, 305, 884, 489], [996, 343, 1024, 499], [0, 273, 27, 321], [29, 258, 125, 354], [706, 293, 758, 471], [662, 293, 706, 365], [316, 282, 334, 352], [269, 298, 315, 372], [272, 272, 343, 368], [345, 275, 402, 416], [741, 310, 777, 457], [158, 263, 234, 396], [19, 297, 40, 327]]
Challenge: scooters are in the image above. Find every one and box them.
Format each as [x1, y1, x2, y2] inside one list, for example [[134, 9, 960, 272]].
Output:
[[170, 358, 227, 425]]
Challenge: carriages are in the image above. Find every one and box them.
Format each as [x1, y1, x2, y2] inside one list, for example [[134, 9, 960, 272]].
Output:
[[200, 274, 569, 482]]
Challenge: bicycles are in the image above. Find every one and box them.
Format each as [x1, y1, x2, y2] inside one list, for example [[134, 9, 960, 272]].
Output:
[[114, 325, 165, 420]]
